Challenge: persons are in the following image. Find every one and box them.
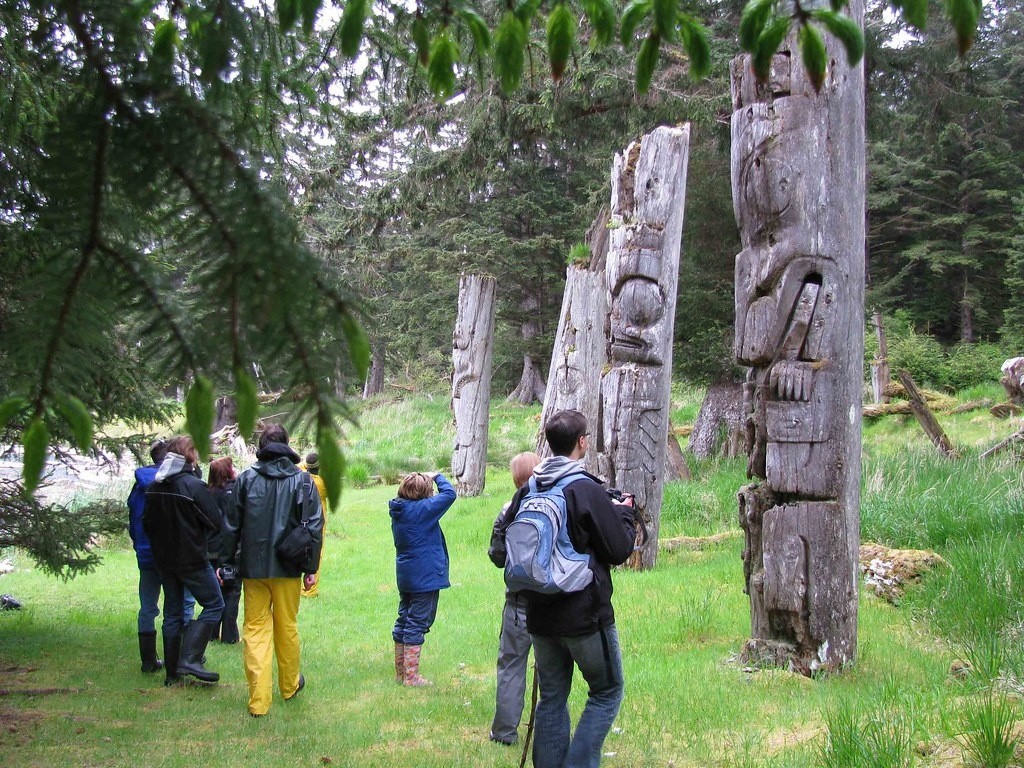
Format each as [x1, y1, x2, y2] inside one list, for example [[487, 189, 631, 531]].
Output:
[[389, 473, 457, 686], [127, 420, 328, 719], [489, 451, 546, 744], [486, 409, 639, 767]]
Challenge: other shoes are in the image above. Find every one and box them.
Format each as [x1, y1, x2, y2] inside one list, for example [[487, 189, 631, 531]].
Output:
[[251, 713, 262, 718], [284, 674, 305, 701]]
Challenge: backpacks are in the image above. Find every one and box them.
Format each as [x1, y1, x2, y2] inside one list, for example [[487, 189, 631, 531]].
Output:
[[502, 474, 596, 595]]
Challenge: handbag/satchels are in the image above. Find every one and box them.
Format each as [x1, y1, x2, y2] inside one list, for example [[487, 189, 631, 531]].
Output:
[[275, 472, 314, 572]]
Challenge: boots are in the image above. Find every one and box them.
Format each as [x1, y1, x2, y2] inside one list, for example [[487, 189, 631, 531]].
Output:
[[198, 655, 208, 663], [393, 641, 405, 684], [402, 643, 433, 686], [175, 619, 221, 682], [163, 636, 185, 685], [137, 630, 162, 671]]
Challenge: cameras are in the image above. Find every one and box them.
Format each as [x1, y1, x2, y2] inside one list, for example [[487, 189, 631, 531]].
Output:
[[219, 567, 238, 593], [607, 488, 626, 503]]
